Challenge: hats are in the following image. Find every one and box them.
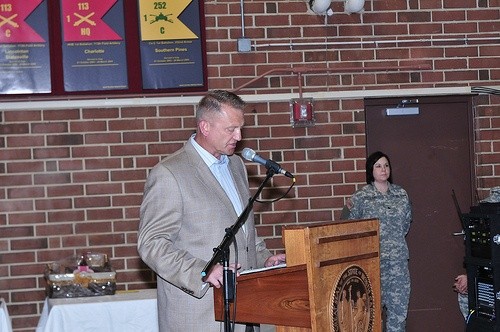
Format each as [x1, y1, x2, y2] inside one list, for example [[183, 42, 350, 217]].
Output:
[[480, 186, 500, 202]]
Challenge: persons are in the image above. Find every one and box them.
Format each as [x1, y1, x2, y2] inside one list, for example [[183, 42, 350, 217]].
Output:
[[136, 89, 288, 331], [451, 185, 499, 323], [339, 150, 414, 331]]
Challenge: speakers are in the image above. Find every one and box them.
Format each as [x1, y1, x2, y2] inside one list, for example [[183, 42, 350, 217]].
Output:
[[461, 202, 500, 332]]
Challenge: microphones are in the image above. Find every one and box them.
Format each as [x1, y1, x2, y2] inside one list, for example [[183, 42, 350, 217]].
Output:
[[241, 146, 294, 179]]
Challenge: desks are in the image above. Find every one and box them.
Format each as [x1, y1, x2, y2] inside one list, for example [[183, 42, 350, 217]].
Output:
[[35, 288, 160, 332]]
